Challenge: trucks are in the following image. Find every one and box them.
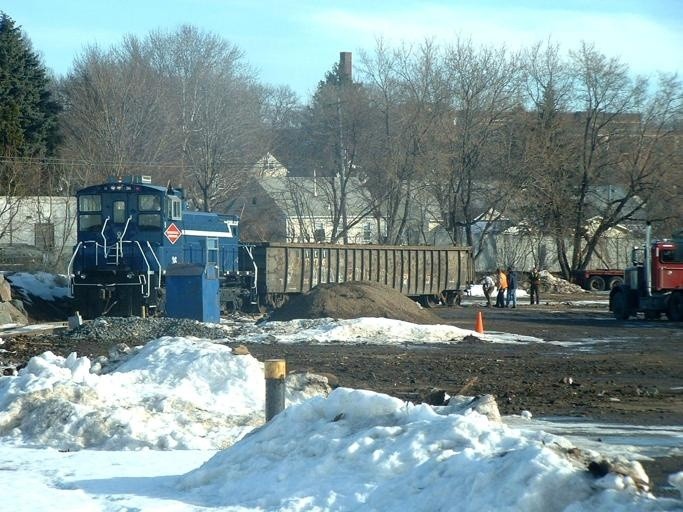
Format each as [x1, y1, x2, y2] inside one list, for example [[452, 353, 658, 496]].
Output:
[[609, 221, 682, 323], [586, 268, 625, 291]]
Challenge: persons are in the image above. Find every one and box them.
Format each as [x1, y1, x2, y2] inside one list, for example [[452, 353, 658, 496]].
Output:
[[505, 267, 517, 308], [493, 268, 508, 308], [481, 274, 494, 308], [529, 269, 541, 305]]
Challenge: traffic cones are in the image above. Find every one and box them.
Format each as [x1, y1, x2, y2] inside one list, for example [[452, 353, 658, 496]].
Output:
[[475, 311, 483, 334]]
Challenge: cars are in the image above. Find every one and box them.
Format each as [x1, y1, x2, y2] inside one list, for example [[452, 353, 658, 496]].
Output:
[[0, 243, 55, 271]]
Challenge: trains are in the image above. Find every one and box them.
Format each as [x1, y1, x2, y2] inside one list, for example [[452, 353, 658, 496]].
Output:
[[68, 180, 475, 317]]
